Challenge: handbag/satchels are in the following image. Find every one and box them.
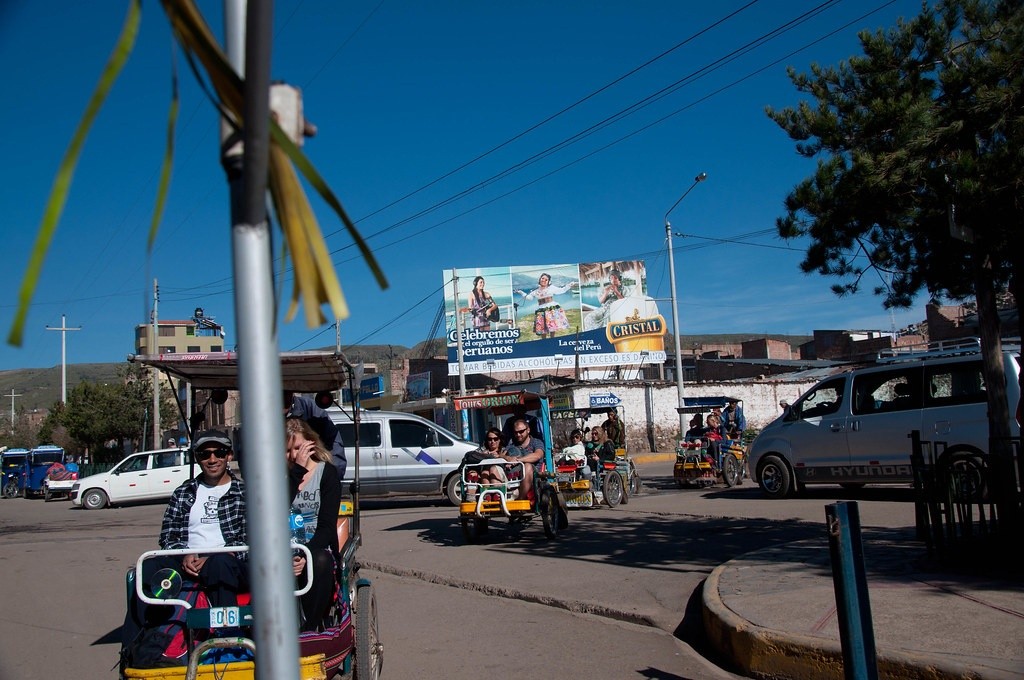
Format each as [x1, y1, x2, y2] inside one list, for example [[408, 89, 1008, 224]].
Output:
[[458, 451, 505, 474], [200, 630, 255, 666]]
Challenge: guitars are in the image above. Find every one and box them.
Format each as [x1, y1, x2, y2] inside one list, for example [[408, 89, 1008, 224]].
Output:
[[459, 306, 500, 322]]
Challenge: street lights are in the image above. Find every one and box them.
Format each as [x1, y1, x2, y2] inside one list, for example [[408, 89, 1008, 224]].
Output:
[[665, 172, 708, 439]]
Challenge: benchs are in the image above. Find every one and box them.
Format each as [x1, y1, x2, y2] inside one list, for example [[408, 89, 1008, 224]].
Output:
[[556, 453, 586, 484]]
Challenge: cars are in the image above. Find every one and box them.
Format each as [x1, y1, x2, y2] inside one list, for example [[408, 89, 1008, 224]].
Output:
[[70, 448, 203, 510]]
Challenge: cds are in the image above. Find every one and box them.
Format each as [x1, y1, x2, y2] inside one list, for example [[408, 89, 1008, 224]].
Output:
[[151, 568, 182, 599]]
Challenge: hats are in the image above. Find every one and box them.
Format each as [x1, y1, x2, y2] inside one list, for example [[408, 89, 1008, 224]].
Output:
[[193, 429, 232, 452], [780, 400, 787, 405]]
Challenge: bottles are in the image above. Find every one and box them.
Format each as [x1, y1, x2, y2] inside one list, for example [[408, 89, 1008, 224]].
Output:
[[288, 508, 306, 555]]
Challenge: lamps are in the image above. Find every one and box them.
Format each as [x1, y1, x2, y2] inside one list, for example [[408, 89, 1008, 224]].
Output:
[[486, 359, 494, 388], [554, 354, 563, 377], [635, 350, 649, 380]]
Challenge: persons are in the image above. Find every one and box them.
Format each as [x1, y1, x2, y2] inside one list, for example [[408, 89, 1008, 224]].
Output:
[[682, 402, 746, 462], [120, 429, 251, 636], [284, 417, 342, 632], [817, 379, 844, 414], [779, 399, 792, 413], [164, 437, 177, 465], [466, 427, 501, 502], [46, 458, 65, 474], [554, 425, 615, 491], [282, 390, 347, 481], [490, 419, 545, 500], [584, 270, 629, 331], [468, 276, 498, 332], [513, 273, 575, 339], [65, 455, 79, 472], [601, 409, 625, 449], [501, 404, 542, 447]]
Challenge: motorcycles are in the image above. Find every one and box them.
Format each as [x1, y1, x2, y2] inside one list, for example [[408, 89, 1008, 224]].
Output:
[[0, 445, 78, 501]]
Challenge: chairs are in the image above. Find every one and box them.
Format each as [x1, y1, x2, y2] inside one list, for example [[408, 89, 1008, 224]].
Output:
[[892, 383, 911, 406]]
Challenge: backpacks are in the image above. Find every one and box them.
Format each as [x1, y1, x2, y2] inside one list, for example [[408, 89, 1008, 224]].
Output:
[[124, 580, 213, 666]]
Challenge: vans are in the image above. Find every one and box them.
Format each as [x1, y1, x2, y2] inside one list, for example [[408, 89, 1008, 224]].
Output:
[[326, 411, 482, 507], [746, 336, 1024, 499]]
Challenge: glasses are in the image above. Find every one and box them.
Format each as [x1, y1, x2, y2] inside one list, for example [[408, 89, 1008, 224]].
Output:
[[488, 437, 499, 441], [592, 432, 598, 436], [283, 398, 294, 420], [513, 429, 526, 434], [571, 435, 579, 439], [195, 448, 230, 460]]
